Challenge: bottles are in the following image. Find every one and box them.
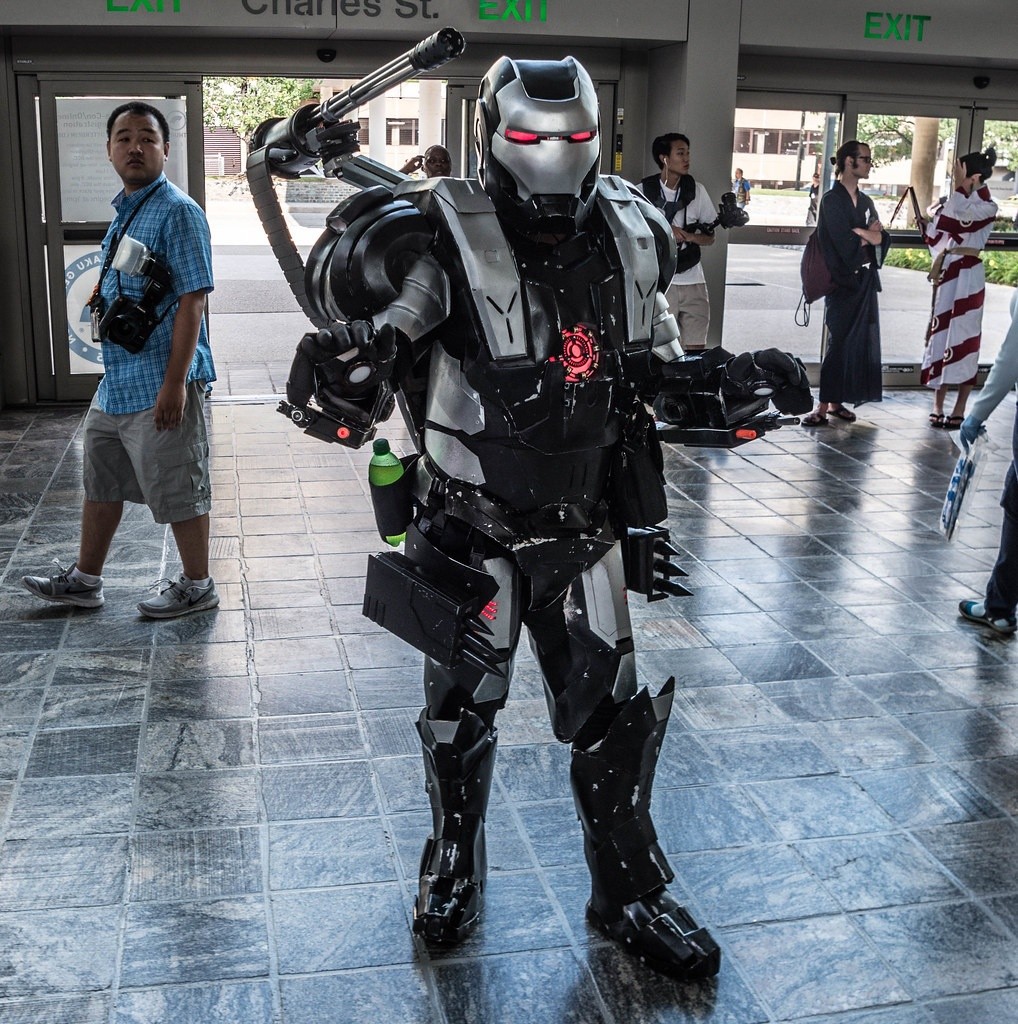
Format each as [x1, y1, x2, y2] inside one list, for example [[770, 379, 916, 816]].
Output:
[[368, 438, 407, 546]]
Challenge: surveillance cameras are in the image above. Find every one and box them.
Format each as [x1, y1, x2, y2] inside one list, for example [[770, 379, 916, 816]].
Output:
[[317, 49, 337, 63], [974, 76, 990, 89]]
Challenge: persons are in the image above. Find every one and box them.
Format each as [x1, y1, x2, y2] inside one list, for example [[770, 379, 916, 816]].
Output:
[[733, 167, 751, 209], [275, 58, 815, 976], [18, 96, 219, 616], [957, 283, 1018, 632], [399, 144, 453, 179], [805, 172, 821, 225], [631, 130, 719, 351], [801, 139, 892, 427], [913, 149, 999, 431]]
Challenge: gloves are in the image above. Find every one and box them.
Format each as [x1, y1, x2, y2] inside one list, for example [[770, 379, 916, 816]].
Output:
[[960, 414, 986, 447]]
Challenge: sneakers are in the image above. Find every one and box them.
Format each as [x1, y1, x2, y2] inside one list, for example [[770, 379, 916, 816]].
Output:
[[22, 558, 104, 607], [137, 573, 220, 617]]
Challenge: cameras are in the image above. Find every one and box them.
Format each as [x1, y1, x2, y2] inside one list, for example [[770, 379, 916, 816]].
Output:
[[97, 234, 172, 354], [718, 192, 750, 229]]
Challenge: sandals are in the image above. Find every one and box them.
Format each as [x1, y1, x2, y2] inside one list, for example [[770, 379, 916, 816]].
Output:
[[959, 601, 1017, 633]]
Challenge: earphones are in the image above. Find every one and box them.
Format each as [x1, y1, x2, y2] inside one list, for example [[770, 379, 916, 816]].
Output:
[[664, 158, 667, 164]]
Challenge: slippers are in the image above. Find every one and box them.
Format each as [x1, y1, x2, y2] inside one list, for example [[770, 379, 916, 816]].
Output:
[[945, 416, 964, 428], [929, 413, 945, 427], [802, 413, 829, 426], [826, 407, 856, 421]]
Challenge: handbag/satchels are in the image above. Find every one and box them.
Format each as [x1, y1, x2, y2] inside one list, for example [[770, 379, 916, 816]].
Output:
[[800, 229, 838, 303], [941, 430, 999, 540]]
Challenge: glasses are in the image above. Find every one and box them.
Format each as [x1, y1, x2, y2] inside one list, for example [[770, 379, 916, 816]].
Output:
[[852, 156, 871, 163]]
